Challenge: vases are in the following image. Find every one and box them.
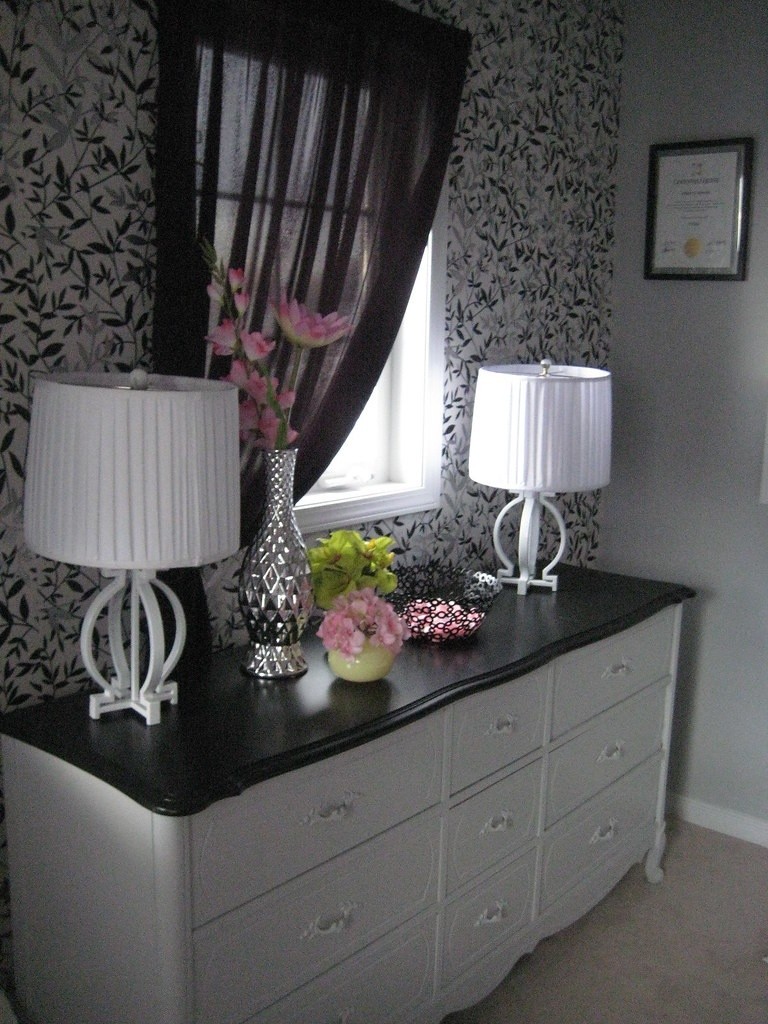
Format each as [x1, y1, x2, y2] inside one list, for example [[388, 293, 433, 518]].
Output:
[[240, 449, 314, 677], [329, 638, 395, 682]]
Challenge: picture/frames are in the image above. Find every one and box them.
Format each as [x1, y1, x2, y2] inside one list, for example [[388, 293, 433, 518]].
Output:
[[642, 129, 754, 281]]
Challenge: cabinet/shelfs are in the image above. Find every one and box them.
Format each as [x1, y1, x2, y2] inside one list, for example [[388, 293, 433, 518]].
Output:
[[0, 560, 695, 1024]]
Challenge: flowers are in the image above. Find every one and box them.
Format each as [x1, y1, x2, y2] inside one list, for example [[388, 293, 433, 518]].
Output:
[[309, 530, 412, 655], [199, 236, 354, 449]]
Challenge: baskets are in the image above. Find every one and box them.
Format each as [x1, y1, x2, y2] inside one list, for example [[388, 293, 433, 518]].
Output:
[[375, 563, 504, 644]]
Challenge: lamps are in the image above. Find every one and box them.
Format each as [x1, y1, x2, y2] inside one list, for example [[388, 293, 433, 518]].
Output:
[[468, 359, 613, 594], [24, 368, 240, 726]]
